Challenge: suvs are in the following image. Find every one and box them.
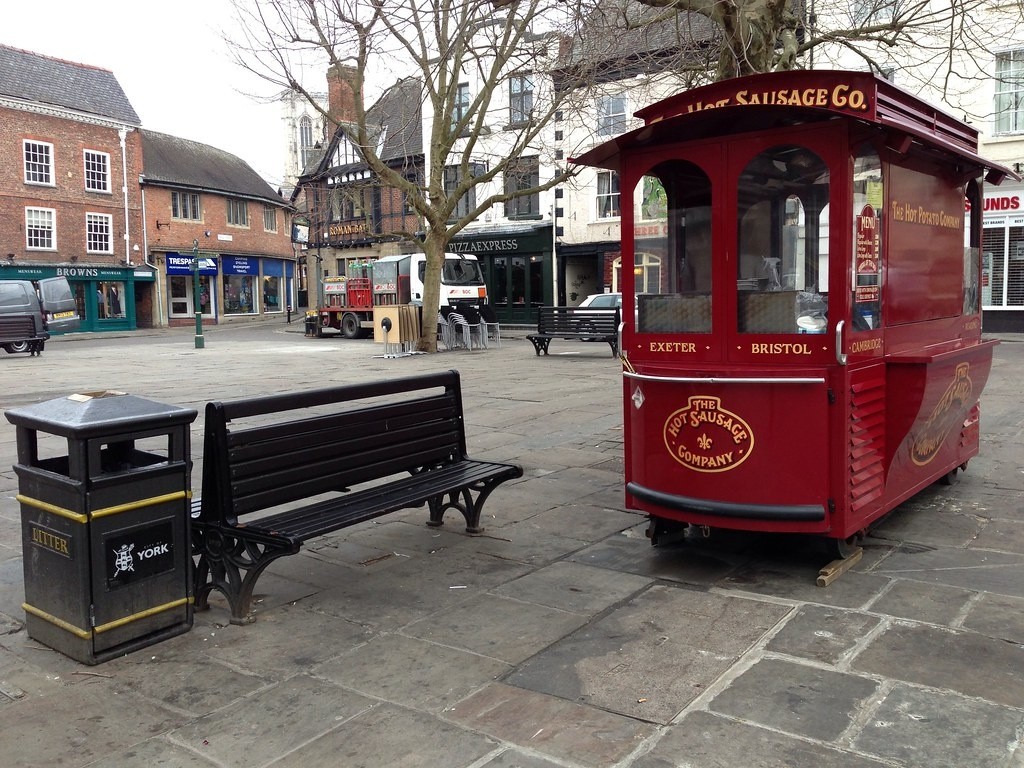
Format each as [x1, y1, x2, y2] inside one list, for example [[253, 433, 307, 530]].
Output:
[[0, 276, 79, 354]]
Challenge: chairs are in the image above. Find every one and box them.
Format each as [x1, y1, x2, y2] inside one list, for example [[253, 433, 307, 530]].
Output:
[[437, 302, 501, 352]]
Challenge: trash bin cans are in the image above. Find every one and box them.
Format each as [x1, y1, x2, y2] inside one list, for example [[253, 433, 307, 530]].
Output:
[[4, 388, 199, 668]]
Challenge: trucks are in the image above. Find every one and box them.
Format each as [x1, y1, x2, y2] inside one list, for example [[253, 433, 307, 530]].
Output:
[[320, 253, 489, 339]]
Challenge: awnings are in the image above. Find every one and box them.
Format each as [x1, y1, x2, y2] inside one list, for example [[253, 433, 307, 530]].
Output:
[[423, 219, 553, 237], [0, 260, 131, 269]]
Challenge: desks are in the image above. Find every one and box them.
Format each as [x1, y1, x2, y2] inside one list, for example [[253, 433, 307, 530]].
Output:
[[372, 304, 427, 358]]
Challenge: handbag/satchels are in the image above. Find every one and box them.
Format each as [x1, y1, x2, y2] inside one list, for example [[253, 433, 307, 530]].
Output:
[[43, 324, 50, 340]]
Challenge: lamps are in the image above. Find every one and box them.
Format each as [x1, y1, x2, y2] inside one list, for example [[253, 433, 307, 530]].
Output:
[[6, 253, 15, 260], [634, 74, 647, 84], [70, 256, 78, 263], [205, 231, 211, 237], [119, 259, 126, 265]]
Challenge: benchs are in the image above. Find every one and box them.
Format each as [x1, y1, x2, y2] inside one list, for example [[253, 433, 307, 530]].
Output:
[[188, 370, 523, 624], [525, 306, 622, 359]]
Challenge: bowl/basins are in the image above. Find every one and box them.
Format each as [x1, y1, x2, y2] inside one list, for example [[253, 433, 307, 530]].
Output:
[[737, 280, 758, 290]]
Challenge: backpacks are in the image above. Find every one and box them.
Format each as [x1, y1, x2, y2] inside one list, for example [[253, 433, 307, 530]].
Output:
[[200, 294, 207, 305]]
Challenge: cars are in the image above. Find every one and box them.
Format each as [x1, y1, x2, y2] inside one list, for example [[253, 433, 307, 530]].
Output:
[[571, 293, 645, 342]]
